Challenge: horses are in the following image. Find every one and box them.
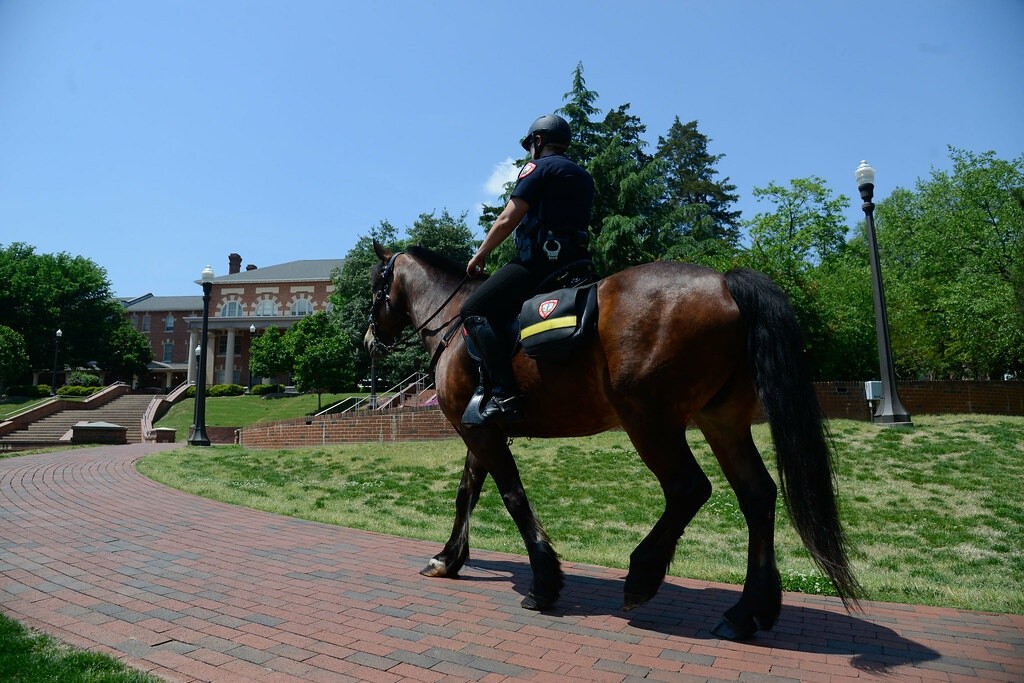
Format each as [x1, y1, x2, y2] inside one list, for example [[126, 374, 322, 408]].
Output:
[[361, 237, 874, 633]]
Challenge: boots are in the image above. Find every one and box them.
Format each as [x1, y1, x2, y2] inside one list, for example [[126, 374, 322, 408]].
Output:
[[463, 316, 527, 426]]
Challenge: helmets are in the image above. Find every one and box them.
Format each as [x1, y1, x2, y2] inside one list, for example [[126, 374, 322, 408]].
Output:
[[522, 115, 572, 152]]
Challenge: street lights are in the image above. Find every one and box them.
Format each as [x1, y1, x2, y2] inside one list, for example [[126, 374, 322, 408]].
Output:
[[49, 328, 62, 396], [244, 324, 256, 394], [850, 160, 914, 426], [186, 264, 215, 445]]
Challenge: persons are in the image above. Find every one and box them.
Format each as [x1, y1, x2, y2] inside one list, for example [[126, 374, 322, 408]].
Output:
[[457, 114, 599, 426]]
